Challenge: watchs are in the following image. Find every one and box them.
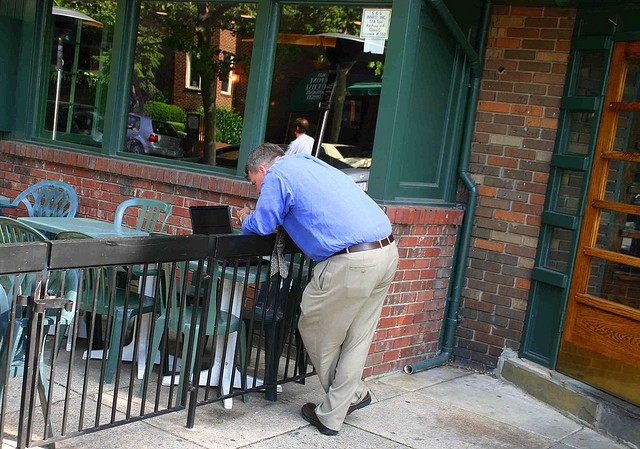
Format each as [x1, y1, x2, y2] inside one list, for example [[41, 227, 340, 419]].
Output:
[[238, 212, 245, 224]]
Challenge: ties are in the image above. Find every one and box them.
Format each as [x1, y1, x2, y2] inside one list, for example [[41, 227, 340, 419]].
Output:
[[270, 225, 289, 279]]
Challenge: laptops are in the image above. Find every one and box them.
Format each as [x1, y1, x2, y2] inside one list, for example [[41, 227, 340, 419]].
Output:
[[188, 205, 274, 268]]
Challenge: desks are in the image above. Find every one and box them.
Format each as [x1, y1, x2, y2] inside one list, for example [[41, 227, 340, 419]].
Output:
[[16, 215, 150, 352], [178, 249, 318, 402]]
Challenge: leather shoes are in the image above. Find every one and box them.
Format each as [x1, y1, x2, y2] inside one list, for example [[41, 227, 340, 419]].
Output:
[[300, 402, 338, 435], [346, 390, 372, 415]]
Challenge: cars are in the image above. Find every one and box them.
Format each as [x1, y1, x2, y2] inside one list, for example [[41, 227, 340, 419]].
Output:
[[177, 143, 370, 194], [309, 137, 375, 167]]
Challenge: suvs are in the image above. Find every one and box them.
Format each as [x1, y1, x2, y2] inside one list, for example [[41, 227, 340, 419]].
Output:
[[126, 112, 183, 157]]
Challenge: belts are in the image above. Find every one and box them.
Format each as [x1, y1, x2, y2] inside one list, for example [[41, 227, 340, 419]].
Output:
[[335, 233, 395, 254]]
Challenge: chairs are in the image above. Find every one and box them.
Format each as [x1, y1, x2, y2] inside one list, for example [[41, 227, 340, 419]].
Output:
[[139, 231, 252, 409], [12, 177, 79, 221], [0, 215, 76, 449], [52, 230, 171, 375], [90, 196, 173, 275], [242, 254, 313, 387]]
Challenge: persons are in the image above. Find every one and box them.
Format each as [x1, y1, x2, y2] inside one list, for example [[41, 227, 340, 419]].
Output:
[[284, 117, 316, 155], [235, 142, 399, 436]]
